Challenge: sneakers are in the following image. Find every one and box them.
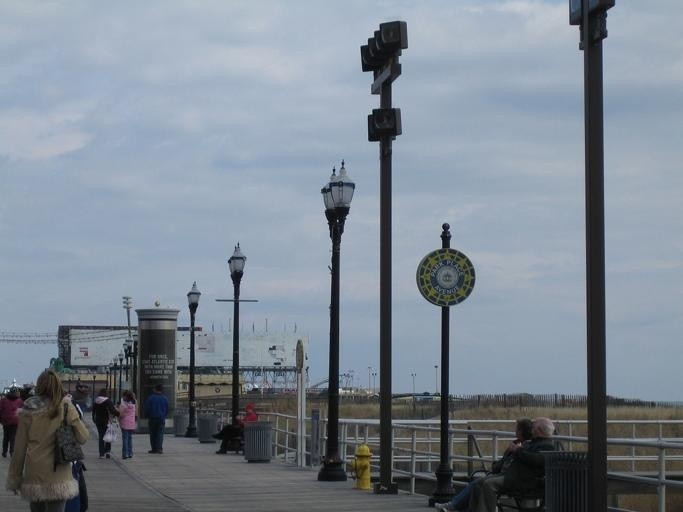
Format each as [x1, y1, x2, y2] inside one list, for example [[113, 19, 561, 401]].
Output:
[[148, 449, 164, 454], [2, 451, 15, 458], [434, 501, 454, 512], [99, 453, 134, 459]]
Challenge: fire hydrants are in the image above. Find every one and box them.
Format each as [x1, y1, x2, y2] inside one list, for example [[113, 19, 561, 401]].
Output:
[[351, 442, 373, 492]]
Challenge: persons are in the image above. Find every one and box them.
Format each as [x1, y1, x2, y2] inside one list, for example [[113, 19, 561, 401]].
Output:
[[114, 390, 135, 460], [92, 388, 119, 460], [466, 417, 556, 512], [142, 384, 168, 454], [212, 403, 257, 454], [435, 418, 533, 512], [6, 369, 88, 512], [0, 385, 36, 458]]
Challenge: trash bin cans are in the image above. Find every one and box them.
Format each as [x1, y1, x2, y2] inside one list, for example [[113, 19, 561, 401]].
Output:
[[173, 414, 188, 436], [242, 421, 273, 463], [198, 415, 217, 443], [538, 450, 589, 512]]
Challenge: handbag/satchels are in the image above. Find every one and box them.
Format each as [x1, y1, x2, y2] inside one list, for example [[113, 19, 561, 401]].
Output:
[[53, 423, 85, 466]]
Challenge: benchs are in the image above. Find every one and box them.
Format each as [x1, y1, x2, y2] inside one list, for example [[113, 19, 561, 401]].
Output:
[[227, 437, 246, 456], [467, 440, 565, 511]]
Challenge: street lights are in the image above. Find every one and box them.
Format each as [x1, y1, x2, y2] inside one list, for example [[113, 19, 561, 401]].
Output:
[[101, 294, 137, 405], [318, 159, 357, 484], [358, 22, 410, 493], [567, 1, 615, 512], [433, 362, 439, 394], [225, 241, 245, 427], [370, 370, 376, 394], [187, 279, 201, 438], [411, 371, 417, 392]]
